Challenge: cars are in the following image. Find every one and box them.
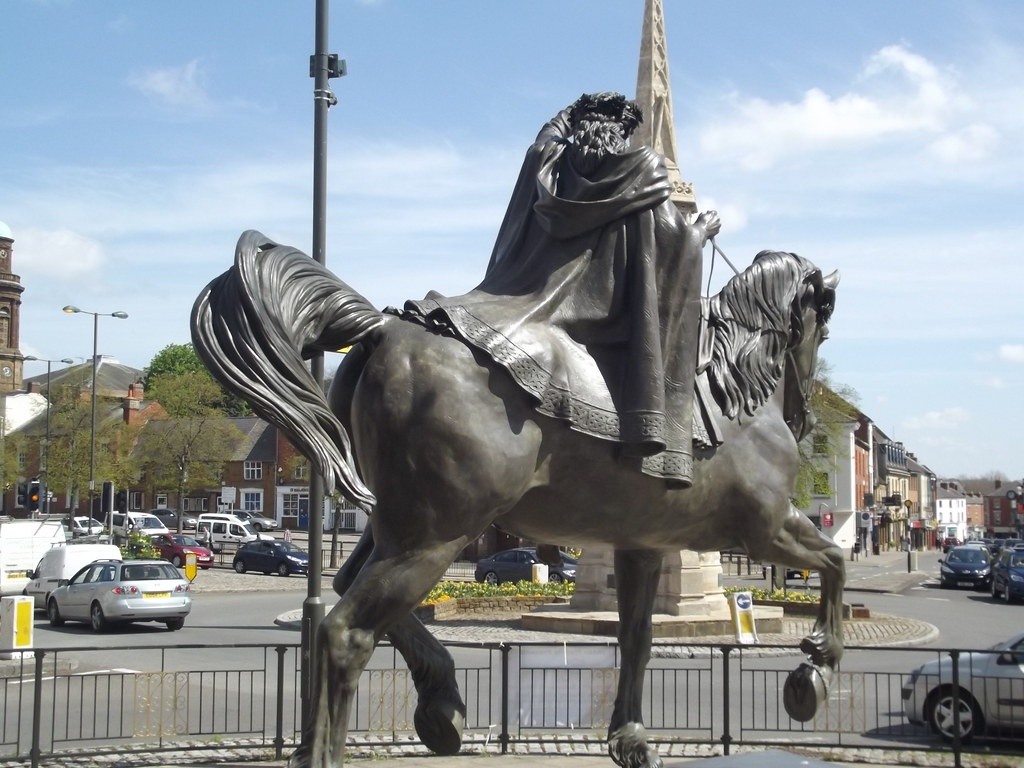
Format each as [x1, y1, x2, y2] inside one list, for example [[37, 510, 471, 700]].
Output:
[[222, 509, 278, 531], [967, 538, 1023, 559], [232, 537, 308, 577], [475, 546, 577, 585], [899, 632, 1023, 747], [148, 534, 214, 569], [939, 546, 994, 590], [761, 564, 807, 581], [148, 508, 197, 529]]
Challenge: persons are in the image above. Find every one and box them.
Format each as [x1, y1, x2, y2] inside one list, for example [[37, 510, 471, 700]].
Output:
[[381, 91, 721, 491]]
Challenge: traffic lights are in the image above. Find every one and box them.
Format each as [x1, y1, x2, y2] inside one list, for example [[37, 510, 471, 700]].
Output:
[[26, 482, 41, 511], [18, 483, 27, 507]]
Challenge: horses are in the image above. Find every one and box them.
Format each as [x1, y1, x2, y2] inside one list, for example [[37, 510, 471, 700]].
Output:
[[190, 230, 847, 768]]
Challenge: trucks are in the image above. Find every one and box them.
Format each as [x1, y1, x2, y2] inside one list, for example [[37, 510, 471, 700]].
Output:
[[0, 518, 66, 597]]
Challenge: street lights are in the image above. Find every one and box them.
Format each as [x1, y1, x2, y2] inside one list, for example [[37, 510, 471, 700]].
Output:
[[62, 306, 127, 533], [300, 52, 347, 753], [819, 503, 829, 530], [25, 354, 72, 514], [904, 500, 913, 573]]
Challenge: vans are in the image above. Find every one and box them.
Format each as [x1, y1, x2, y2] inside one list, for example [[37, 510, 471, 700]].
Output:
[[103, 510, 170, 547], [195, 520, 275, 553], [23, 543, 123, 615], [199, 513, 250, 525]]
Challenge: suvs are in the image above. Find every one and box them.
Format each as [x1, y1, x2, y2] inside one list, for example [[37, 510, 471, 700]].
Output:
[[943, 538, 961, 552], [991, 546, 1024, 602], [62, 514, 104, 540], [45, 558, 191, 631]]
